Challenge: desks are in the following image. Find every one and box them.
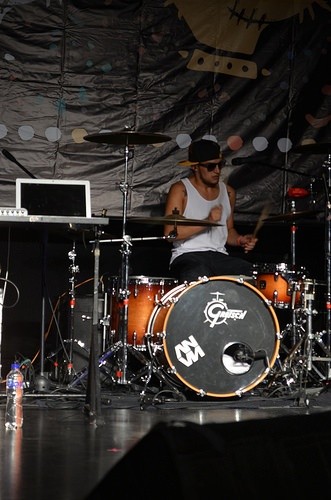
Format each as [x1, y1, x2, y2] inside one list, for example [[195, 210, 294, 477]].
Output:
[[0, 215, 109, 416]]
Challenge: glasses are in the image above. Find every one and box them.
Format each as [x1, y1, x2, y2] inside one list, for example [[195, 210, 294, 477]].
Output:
[[197, 157, 224, 172]]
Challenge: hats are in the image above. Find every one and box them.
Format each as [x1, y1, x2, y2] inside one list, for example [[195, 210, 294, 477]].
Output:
[[188, 139, 221, 163]]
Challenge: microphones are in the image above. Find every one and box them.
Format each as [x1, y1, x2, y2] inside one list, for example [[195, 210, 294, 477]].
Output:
[[231, 158, 255, 165]]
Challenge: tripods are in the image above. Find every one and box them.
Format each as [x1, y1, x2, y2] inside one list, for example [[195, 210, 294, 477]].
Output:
[[61, 137, 180, 397], [265, 220, 331, 396]]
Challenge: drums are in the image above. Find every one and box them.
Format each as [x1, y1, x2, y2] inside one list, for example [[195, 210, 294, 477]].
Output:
[[147, 277, 282, 398], [252, 263, 305, 307], [110, 274, 180, 352]]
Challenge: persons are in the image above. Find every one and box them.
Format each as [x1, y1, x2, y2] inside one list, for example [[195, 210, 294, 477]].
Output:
[[164, 139, 258, 283]]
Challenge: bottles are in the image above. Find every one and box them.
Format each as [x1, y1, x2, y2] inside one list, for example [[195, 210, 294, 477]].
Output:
[[4, 364, 23, 430]]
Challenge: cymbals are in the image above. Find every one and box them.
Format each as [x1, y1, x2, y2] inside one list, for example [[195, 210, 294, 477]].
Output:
[[261, 209, 317, 222], [83, 129, 173, 145], [127, 211, 226, 227]]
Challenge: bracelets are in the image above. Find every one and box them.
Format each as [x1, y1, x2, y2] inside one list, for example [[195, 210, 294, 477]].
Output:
[[237, 235, 243, 246]]
